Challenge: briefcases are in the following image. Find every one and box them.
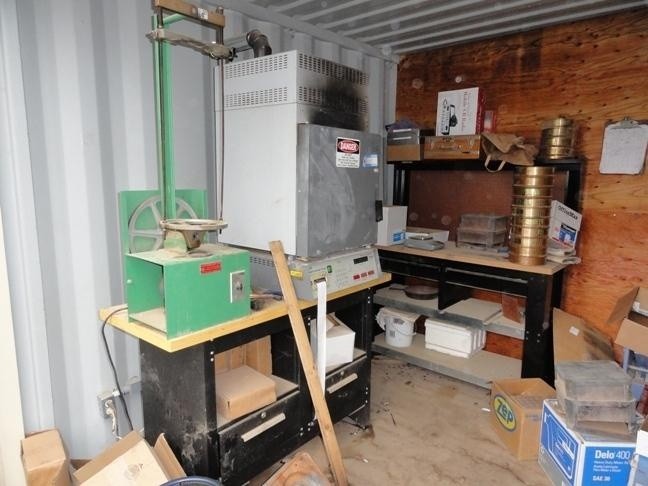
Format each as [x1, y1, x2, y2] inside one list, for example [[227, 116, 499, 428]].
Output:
[[423, 135, 486, 159]]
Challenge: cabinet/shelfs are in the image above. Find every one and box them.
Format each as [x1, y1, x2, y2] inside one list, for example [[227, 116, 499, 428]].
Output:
[[132, 288, 373, 486]]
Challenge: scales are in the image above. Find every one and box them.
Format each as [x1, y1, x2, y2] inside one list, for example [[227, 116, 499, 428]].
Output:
[[404, 235, 445, 251]]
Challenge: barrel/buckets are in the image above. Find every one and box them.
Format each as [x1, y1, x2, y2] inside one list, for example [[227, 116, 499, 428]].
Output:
[[385, 317, 417, 348]]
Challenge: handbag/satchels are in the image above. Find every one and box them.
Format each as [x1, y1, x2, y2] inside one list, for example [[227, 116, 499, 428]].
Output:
[[482, 131, 539, 167]]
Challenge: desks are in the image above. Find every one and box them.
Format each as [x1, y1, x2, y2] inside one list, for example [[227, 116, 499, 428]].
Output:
[[359, 246, 567, 389]]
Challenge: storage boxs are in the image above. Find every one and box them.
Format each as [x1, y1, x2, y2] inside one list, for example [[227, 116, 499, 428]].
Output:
[[488, 286, 647, 483], [19, 426, 189, 485]]
